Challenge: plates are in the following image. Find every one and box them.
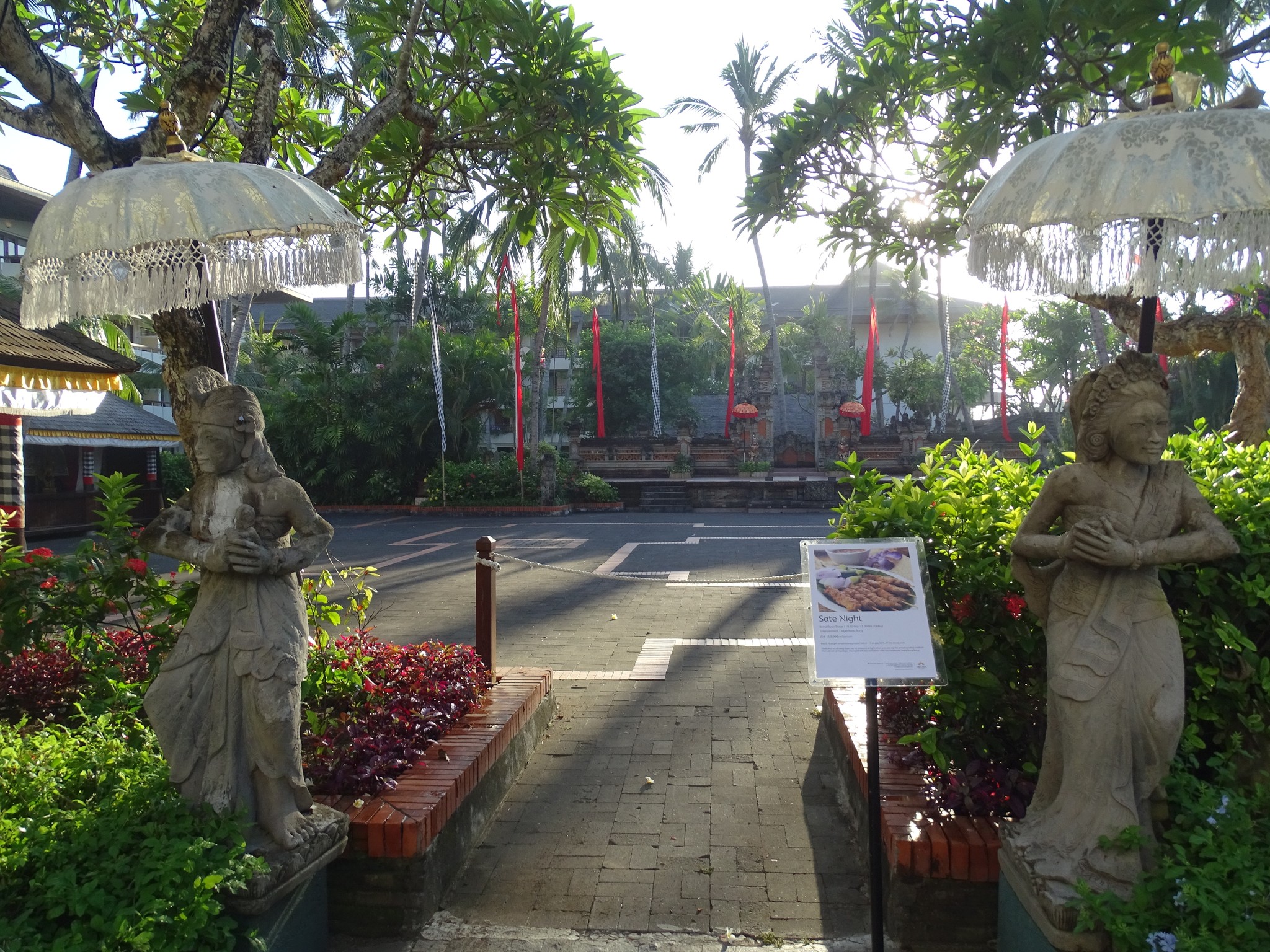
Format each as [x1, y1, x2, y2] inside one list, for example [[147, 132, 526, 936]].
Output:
[[815, 566, 918, 612]]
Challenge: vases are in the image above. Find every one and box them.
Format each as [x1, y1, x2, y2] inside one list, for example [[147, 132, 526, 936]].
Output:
[[670, 473, 691, 478]]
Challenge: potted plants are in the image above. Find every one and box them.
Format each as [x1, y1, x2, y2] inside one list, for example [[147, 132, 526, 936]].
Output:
[[824, 459, 846, 477], [738, 460, 771, 477]]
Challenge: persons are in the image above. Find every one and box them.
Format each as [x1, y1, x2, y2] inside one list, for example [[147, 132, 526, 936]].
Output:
[[138, 385, 334, 851], [1011, 349, 1239, 899]]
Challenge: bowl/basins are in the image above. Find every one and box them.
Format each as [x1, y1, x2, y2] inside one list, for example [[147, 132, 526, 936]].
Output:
[[825, 549, 871, 565]]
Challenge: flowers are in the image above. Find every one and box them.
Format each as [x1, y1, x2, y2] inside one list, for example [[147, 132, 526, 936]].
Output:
[[667, 454, 692, 473]]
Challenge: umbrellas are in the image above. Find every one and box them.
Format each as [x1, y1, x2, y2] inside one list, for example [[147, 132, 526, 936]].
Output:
[[955, 41, 1269, 353], [839, 401, 866, 457], [731, 402, 759, 462], [18, 99, 365, 382]]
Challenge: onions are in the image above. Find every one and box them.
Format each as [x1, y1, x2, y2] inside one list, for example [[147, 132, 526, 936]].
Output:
[[816, 567, 852, 588]]
